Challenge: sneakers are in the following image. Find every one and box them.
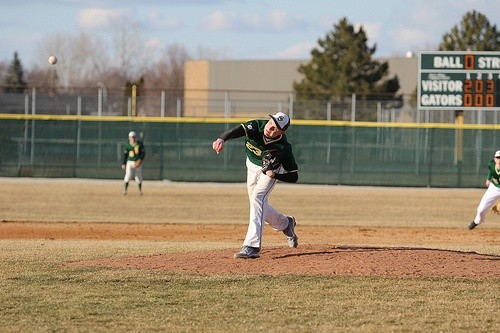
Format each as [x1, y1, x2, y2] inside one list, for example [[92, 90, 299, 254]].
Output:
[[234, 245, 260, 259], [282, 215, 298, 248]]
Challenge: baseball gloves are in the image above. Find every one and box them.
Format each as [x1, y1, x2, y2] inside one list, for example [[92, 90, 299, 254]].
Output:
[[492, 202, 500, 215], [262, 150, 281, 174]]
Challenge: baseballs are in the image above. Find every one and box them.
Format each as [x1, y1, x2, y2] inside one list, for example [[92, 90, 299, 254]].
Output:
[[405, 51, 415, 60], [49, 56, 57, 64]]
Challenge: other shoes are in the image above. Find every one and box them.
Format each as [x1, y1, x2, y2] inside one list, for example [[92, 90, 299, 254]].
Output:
[[467, 221, 478, 229]]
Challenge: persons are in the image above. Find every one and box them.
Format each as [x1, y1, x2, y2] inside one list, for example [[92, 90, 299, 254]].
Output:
[[468, 149, 500, 230], [122, 130, 145, 196], [212, 111, 299, 259]]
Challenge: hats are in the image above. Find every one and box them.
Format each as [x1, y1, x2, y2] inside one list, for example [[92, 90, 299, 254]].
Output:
[[495, 150, 500, 158], [129, 131, 136, 137], [268, 111, 290, 130]]
